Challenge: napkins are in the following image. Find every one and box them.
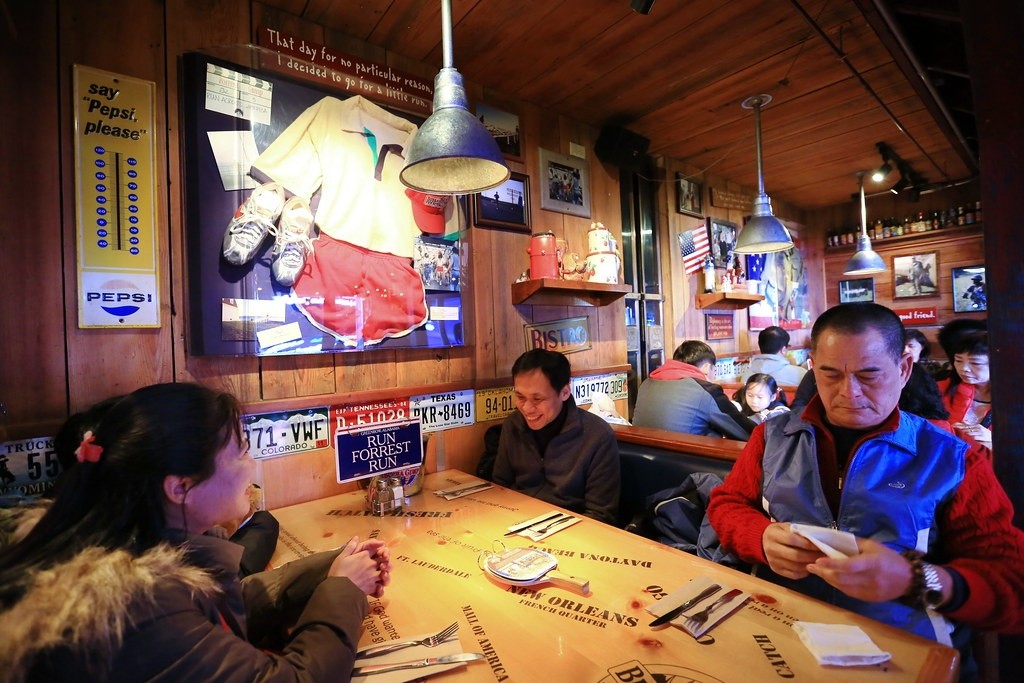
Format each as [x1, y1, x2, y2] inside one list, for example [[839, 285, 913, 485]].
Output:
[[645, 576, 752, 641], [349, 632, 467, 683], [790, 620, 891, 668], [433, 480, 494, 502], [507, 510, 581, 544], [790, 523, 860, 560]]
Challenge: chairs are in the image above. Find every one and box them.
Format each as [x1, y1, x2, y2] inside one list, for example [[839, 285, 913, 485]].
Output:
[[618, 449, 676, 536], [478, 422, 504, 480]]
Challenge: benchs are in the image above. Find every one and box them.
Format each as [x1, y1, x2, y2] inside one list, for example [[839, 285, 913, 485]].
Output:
[[606, 420, 748, 546], [720, 381, 798, 409]]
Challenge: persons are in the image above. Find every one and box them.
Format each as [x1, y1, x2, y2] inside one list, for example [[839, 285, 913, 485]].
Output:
[[903, 330, 940, 376], [908, 257, 924, 295], [492, 347, 621, 524], [707, 300, 1024, 648], [632, 340, 758, 441], [731, 373, 788, 424], [550, 172, 581, 204], [712, 223, 736, 261], [928, 318, 993, 450], [0, 378, 391, 683], [789, 361, 956, 438], [683, 190, 695, 210], [740, 325, 808, 387], [963, 274, 986, 308]]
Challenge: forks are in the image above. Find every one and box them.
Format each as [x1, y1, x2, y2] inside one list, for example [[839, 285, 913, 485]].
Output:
[[683, 589, 743, 634], [354, 622, 459, 660], [527, 516, 575, 533], [441, 485, 492, 495]]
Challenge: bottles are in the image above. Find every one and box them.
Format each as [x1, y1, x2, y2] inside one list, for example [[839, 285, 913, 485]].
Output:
[[527, 233, 560, 281], [704, 257, 716, 293], [375, 477, 403, 511]]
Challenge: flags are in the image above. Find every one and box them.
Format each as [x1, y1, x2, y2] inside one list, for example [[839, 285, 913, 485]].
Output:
[[677, 224, 711, 276]]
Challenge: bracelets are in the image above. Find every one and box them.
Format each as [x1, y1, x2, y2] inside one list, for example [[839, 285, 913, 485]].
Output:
[[901, 549, 941, 605]]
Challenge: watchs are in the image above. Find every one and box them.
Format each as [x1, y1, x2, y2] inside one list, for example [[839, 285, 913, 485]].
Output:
[[923, 561, 942, 610]]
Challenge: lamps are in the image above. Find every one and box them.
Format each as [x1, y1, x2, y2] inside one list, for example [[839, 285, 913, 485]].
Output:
[[398, 0, 511, 198], [889, 171, 909, 195], [907, 182, 922, 203], [732, 92, 794, 255], [629, 0, 656, 17], [841, 171, 887, 277], [871, 154, 892, 182]]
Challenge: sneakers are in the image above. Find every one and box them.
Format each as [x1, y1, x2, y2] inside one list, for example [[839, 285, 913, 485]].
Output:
[[271, 196, 314, 287], [224, 181, 285, 265]]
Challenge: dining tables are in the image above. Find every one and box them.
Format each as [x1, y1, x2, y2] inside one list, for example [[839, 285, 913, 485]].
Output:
[[258, 467, 961, 683]]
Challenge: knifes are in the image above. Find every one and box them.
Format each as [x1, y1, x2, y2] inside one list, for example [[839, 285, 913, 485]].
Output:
[[504, 514, 563, 536], [351, 653, 486, 677], [436, 483, 491, 496], [649, 584, 721, 627]]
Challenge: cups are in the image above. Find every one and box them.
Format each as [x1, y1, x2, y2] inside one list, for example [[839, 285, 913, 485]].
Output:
[[749, 280, 757, 295]]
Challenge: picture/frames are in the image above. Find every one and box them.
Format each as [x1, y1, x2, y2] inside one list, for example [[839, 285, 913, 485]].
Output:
[[707, 216, 740, 270], [473, 171, 533, 235], [890, 250, 941, 301], [537, 147, 591, 219], [675, 171, 706, 220], [470, 94, 527, 164], [705, 313, 734, 341], [951, 263, 988, 314], [838, 277, 876, 304]]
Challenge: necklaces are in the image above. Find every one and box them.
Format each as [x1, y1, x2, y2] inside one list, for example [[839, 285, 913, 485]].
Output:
[[424, 247, 459, 291]]
